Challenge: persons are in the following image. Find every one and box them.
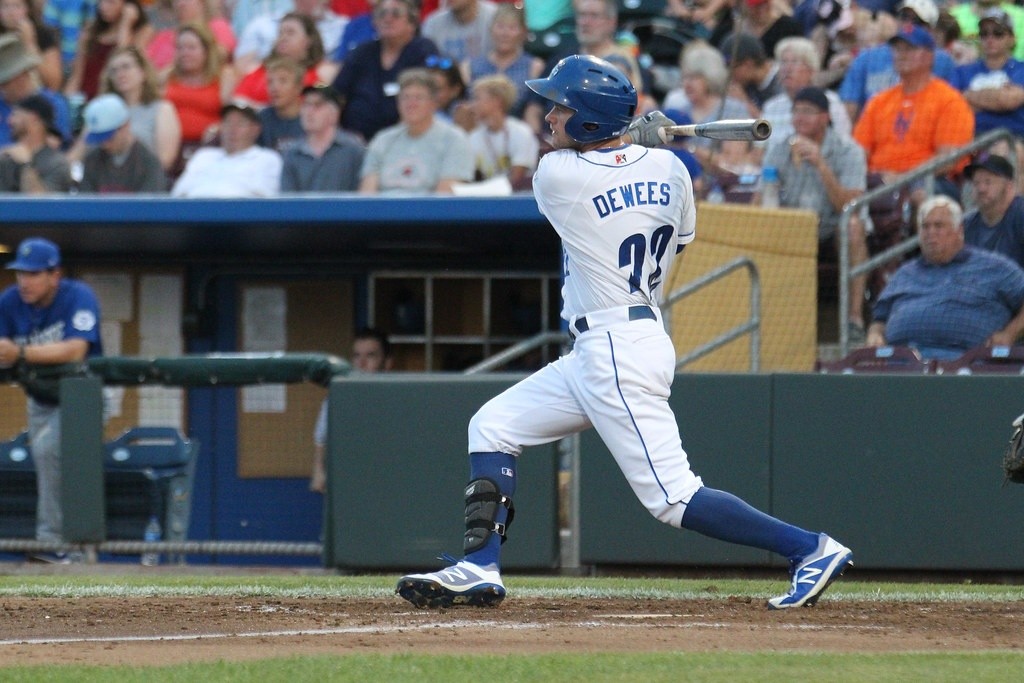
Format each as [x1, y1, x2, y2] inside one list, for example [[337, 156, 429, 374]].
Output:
[[865, 195, 1024, 362], [749, 86, 875, 341], [959, 155, 1024, 266], [0, 237, 102, 563], [0, 0, 1024, 207], [311, 324, 393, 493], [394, 54, 853, 608]]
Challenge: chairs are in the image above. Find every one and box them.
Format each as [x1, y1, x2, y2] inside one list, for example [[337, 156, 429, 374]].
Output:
[[1, 426, 187, 567]]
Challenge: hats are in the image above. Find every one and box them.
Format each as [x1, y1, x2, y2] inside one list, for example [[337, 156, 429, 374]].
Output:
[[978, 4, 1016, 29], [7, 237, 60, 271], [302, 84, 347, 107], [795, 87, 832, 126], [220, 103, 263, 122], [82, 93, 130, 144], [888, 27, 935, 50], [897, 0, 941, 28], [962, 152, 1015, 180]]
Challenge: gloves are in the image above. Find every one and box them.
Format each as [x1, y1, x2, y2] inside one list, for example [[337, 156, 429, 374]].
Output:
[[626, 111, 676, 147]]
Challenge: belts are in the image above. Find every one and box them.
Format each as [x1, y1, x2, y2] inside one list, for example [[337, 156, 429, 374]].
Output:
[[569, 306, 657, 341]]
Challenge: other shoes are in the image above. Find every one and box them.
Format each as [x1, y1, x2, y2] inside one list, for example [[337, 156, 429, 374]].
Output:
[[29, 550, 73, 565]]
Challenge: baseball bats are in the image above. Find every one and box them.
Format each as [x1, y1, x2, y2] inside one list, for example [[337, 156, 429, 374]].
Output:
[[663, 116, 773, 142]]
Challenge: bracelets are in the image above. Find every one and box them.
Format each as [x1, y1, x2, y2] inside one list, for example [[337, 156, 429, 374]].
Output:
[[15, 347, 25, 366]]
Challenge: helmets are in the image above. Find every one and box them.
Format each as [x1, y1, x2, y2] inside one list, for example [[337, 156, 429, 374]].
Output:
[[525, 54, 638, 143]]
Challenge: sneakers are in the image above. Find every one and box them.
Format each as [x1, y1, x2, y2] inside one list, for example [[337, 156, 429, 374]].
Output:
[[396, 552, 507, 610], [767, 532, 855, 610]]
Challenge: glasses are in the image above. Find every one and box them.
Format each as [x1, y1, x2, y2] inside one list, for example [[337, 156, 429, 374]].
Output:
[[979, 28, 1012, 39], [425, 55, 454, 70]]
